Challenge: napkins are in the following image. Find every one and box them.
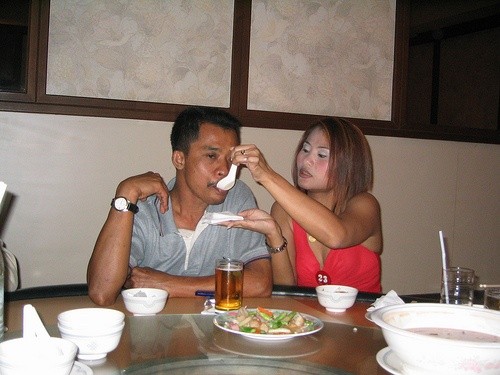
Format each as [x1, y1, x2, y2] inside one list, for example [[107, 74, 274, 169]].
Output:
[[201, 211, 244, 223], [366, 291, 403, 313]]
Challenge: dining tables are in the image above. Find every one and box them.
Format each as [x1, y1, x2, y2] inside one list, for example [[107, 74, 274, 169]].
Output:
[[0, 295, 392, 375]]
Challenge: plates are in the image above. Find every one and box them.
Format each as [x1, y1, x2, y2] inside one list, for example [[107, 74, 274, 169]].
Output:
[[212, 308, 325, 340], [376, 344, 408, 375], [69, 360, 93, 374]]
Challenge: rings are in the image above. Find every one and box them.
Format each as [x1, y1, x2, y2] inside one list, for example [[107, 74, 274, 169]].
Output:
[[241, 150, 245, 155]]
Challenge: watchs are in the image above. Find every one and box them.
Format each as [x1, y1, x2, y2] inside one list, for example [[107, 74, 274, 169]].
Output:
[[111, 196, 139, 213]]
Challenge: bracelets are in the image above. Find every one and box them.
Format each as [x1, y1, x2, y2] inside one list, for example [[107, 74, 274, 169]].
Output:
[[265, 236, 287, 253]]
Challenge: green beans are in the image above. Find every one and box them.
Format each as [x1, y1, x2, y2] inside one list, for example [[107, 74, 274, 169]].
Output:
[[239, 310, 314, 332]]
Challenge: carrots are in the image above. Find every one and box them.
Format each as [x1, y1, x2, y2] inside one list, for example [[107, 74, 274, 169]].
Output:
[[258, 306, 272, 316]]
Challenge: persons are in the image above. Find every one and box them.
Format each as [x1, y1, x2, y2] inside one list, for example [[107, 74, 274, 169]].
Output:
[[230, 116, 383, 292], [87, 106, 272, 306]]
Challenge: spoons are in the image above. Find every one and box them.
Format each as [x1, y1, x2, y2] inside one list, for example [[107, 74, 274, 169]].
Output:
[[217, 164, 237, 190]]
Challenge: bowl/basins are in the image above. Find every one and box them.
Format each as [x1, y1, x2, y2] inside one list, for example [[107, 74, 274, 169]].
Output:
[[121, 288, 168, 316], [364, 303, 500, 375], [316, 284, 358, 312], [0, 337, 94, 375], [57, 308, 126, 360]]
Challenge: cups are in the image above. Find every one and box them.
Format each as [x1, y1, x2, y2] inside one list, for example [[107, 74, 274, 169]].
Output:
[[215, 258, 244, 313], [440, 266, 475, 306], [479, 284, 500, 311]]
